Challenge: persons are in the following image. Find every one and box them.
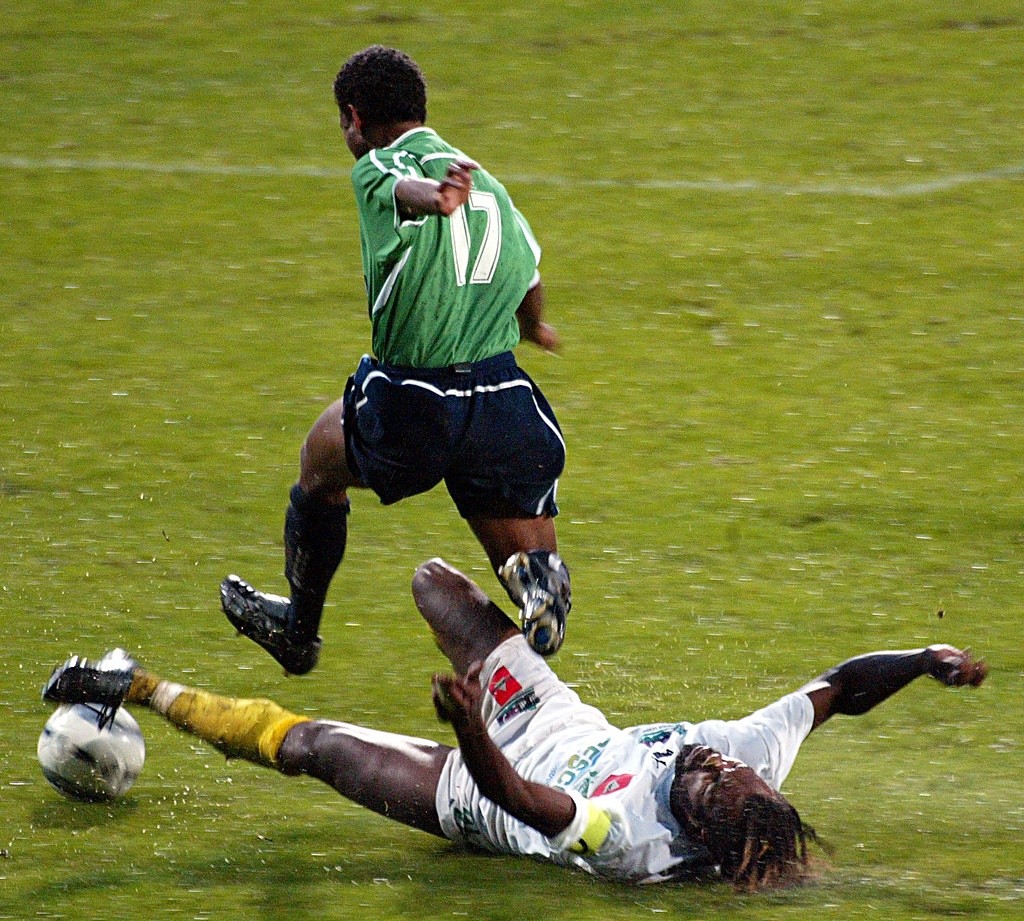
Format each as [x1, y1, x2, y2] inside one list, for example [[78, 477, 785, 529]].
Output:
[[41, 558, 987, 892], [220, 44, 572, 675]]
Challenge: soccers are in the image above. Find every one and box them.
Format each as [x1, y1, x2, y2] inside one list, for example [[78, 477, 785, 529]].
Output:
[[36, 703, 148, 803]]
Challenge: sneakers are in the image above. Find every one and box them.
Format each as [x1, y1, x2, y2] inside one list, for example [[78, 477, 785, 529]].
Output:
[[42, 647, 135, 730], [220, 575, 323, 676], [503, 548, 572, 656]]
